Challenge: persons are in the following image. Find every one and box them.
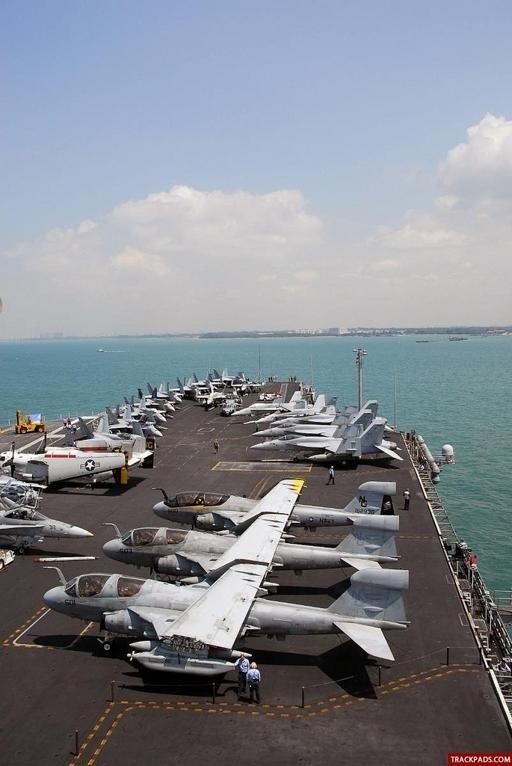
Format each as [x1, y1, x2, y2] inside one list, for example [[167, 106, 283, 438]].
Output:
[[214, 439, 219, 454], [403, 488, 411, 510], [498, 659, 511, 671], [235, 654, 249, 694], [326, 465, 336, 486], [256, 424, 259, 432], [288, 375, 297, 382], [63, 417, 82, 432], [268, 376, 272, 382], [456, 538, 468, 552], [246, 662, 262, 704], [470, 554, 477, 569], [28, 416, 31, 424]]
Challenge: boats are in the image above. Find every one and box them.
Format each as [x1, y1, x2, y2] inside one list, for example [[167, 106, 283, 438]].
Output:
[[448, 335, 471, 342], [415, 339, 429, 342]]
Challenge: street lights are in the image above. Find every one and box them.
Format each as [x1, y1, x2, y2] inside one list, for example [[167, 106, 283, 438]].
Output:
[[352, 346, 368, 409]]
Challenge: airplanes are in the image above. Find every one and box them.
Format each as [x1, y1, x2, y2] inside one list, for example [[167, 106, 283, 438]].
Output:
[[34, 555, 414, 681], [96, 512, 405, 574]]
